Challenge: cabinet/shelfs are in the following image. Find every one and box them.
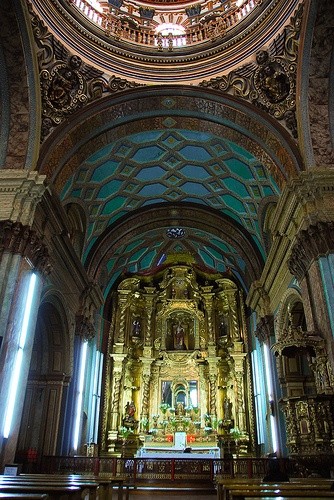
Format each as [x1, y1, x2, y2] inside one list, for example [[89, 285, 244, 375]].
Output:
[[213, 473, 334, 500]]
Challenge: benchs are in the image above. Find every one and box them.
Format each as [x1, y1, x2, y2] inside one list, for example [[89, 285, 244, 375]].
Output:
[[0, 471, 129, 500]]
